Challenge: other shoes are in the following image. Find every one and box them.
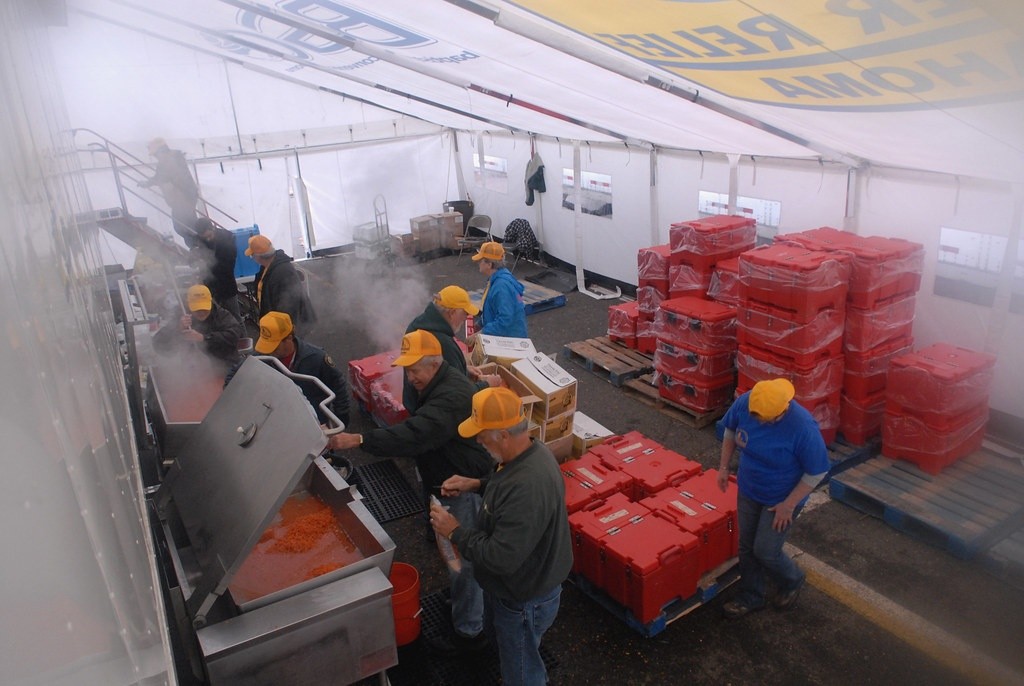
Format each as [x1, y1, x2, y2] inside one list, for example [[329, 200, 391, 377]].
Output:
[[724, 600, 754, 617], [778, 576, 806, 610]]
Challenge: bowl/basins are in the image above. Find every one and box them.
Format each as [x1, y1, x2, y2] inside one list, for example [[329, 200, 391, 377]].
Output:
[[322, 453, 354, 481]]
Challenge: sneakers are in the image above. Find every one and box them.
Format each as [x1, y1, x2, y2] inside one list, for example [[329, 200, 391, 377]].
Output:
[[429, 630, 489, 657]]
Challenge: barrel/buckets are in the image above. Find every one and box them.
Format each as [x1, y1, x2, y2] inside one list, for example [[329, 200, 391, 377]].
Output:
[[388, 562, 423, 646]]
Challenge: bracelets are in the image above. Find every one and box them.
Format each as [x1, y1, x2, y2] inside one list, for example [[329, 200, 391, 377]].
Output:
[[360, 435, 363, 443], [720, 466, 727, 469], [448, 527, 458, 539]]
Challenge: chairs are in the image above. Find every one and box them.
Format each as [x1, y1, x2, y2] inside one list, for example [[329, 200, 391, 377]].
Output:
[[457, 215, 494, 265]]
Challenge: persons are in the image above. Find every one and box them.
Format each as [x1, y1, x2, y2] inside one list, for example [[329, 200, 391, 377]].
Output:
[[327, 329, 498, 656], [430, 387, 574, 686], [153, 285, 240, 361], [464, 242, 527, 346], [402, 285, 502, 545], [717, 377, 831, 619], [137, 138, 199, 247], [244, 235, 317, 340], [188, 217, 239, 314], [223, 311, 352, 455]]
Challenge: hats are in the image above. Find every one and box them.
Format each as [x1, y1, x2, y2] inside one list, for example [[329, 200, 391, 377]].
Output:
[[244, 235, 271, 256], [457, 386, 525, 438], [471, 242, 504, 260], [390, 330, 442, 367], [435, 285, 479, 315], [255, 312, 292, 354], [187, 285, 212, 312], [749, 378, 795, 421]]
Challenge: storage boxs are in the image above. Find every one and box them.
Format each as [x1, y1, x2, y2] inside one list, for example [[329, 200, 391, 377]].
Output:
[[354, 211, 463, 258], [610, 214, 997, 475], [348, 336, 738, 622], [227, 224, 261, 277]]
